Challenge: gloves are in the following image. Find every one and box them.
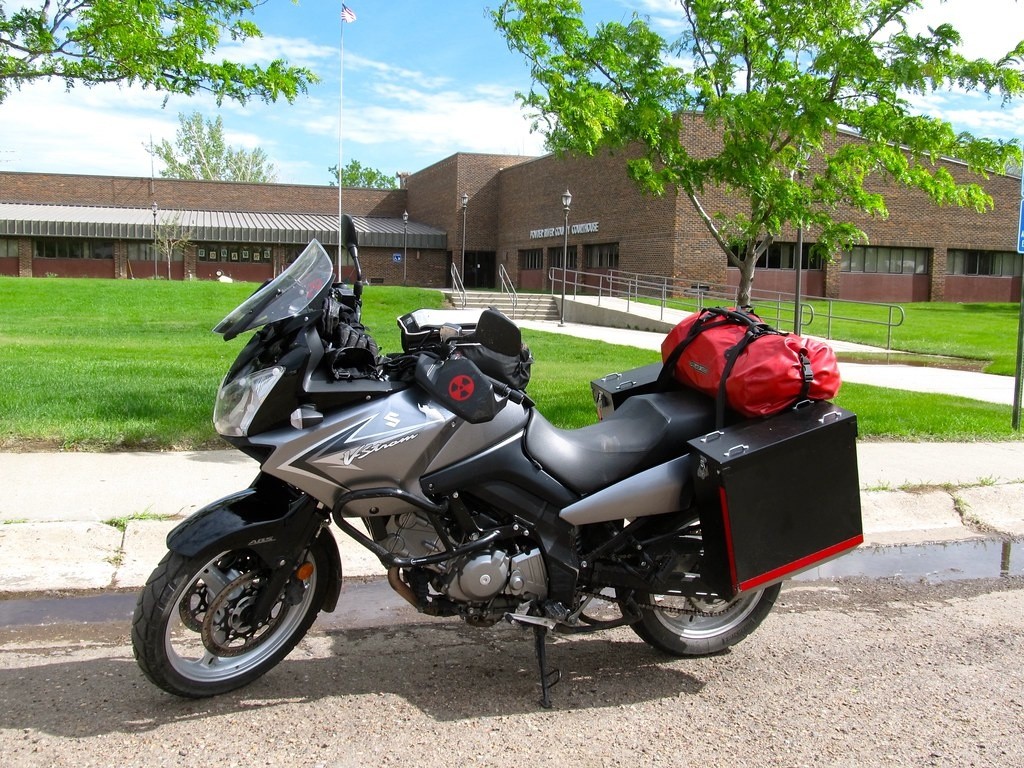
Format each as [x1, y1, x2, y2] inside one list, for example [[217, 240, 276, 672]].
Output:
[[320, 325, 380, 381]]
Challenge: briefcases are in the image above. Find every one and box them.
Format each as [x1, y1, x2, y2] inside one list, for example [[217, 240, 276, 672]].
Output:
[[590, 360, 668, 422], [687, 398, 864, 595]]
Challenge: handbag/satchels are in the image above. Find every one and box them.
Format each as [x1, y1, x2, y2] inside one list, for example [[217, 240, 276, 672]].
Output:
[[660, 304, 841, 420]]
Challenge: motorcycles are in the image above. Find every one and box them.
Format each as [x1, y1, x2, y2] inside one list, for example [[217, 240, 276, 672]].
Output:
[[130, 214, 785, 713]]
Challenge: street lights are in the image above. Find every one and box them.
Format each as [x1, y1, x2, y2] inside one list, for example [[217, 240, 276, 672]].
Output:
[[151, 201, 159, 279], [403, 210, 408, 287], [558, 190, 571, 328], [459, 192, 468, 290]]
